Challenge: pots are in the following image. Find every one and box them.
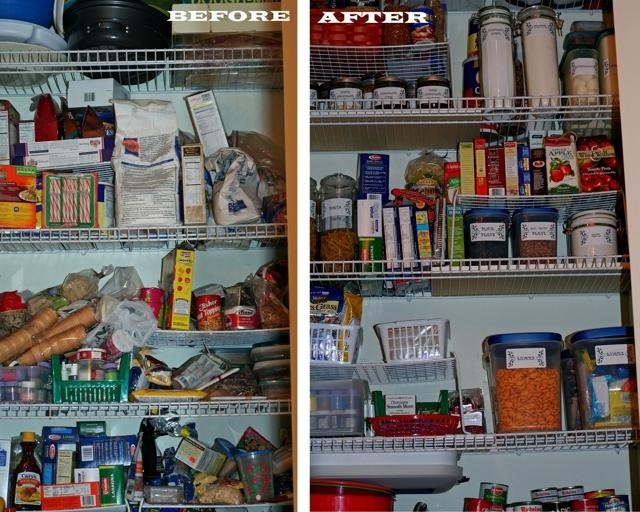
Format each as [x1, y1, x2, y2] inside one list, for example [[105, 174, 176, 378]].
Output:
[[62, 0, 173, 86]]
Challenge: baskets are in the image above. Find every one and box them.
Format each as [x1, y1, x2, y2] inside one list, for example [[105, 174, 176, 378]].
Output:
[[311, 323, 363, 364], [368, 414, 460, 436], [52, 352, 131, 403], [375, 319, 449, 364]]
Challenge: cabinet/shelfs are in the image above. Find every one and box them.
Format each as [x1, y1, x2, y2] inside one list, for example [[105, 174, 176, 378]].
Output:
[[311, 1, 640, 512], [0, 0, 297, 512]]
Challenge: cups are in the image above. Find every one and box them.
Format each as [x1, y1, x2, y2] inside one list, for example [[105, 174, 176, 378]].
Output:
[[236, 448, 275, 503]]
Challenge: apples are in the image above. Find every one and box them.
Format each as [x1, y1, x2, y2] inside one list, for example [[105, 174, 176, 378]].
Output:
[[550, 162, 572, 182], [0, 291, 28, 311]]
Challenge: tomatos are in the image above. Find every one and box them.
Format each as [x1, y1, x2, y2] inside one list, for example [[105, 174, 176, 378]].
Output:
[[580, 141, 619, 192]]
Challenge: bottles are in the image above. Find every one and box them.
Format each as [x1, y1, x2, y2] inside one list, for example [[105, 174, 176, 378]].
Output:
[[10, 432, 43, 510], [479, 6, 558, 109], [310, 75, 455, 111], [307, 174, 356, 272]]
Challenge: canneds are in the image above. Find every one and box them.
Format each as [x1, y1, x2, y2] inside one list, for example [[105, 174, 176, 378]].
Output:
[[462, 16, 485, 107], [224, 306, 259, 330], [140, 287, 165, 329], [195, 293, 222, 328], [98, 181, 114, 236], [461, 482, 630, 512], [309, 4, 451, 110], [64, 330, 133, 381]]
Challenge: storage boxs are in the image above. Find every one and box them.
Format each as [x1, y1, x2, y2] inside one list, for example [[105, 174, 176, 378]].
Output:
[[511, 208, 559, 264], [561, 349, 582, 435], [565, 326, 639, 435], [311, 379, 369, 436], [482, 332, 564, 438], [463, 208, 510, 266]]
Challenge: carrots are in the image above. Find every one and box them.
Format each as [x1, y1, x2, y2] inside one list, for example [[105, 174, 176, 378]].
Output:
[[1, 306, 97, 367]]
